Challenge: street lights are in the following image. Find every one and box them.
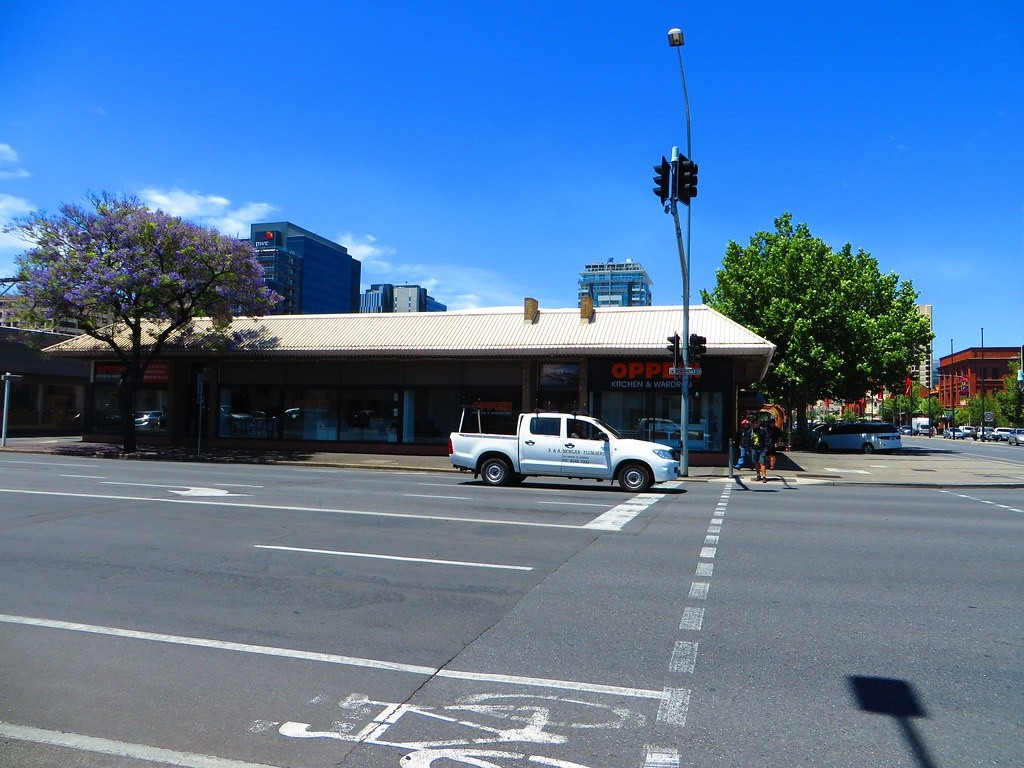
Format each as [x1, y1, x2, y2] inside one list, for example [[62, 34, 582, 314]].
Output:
[[665, 25, 699, 479]]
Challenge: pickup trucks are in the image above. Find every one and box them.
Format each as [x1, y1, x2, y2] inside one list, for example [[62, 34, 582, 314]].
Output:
[[449, 412, 681, 493], [620, 417, 712, 448]]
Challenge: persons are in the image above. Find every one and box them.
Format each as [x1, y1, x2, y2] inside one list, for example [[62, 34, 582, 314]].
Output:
[[783, 418, 788, 432], [591, 427, 609, 441], [733, 416, 783, 483], [570, 421, 589, 438]]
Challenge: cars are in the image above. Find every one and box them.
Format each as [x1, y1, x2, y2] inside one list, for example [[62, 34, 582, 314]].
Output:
[[104, 410, 125, 429], [899, 425, 916, 436], [970, 426, 979, 440], [792, 420, 827, 441], [219, 403, 301, 436], [943, 428, 965, 439], [361, 410, 384, 426], [977, 427, 995, 441], [57, 409, 82, 428], [1009, 428, 1024, 445], [135, 411, 163, 430]]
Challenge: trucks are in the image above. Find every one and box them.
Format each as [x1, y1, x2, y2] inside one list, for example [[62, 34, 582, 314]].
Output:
[[959, 426, 975, 436], [912, 417, 930, 436]]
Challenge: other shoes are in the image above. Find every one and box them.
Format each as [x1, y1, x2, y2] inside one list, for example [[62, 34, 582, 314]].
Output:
[[754, 476, 761, 481], [763, 477, 766, 483], [770, 466, 775, 470], [733, 466, 740, 470]]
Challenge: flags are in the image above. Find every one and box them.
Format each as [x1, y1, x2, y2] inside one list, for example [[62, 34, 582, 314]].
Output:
[[904, 377, 911, 397], [825, 398, 829, 408], [878, 385, 883, 400], [845, 396, 866, 416], [890, 393, 895, 400]]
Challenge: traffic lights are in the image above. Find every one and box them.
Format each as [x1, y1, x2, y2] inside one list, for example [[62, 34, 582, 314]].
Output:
[[689, 333, 708, 365], [675, 153, 699, 206], [666, 331, 680, 367], [653, 156, 669, 205]]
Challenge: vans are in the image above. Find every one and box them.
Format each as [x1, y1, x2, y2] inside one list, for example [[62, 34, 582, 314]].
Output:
[[992, 427, 1014, 442], [815, 422, 902, 454]]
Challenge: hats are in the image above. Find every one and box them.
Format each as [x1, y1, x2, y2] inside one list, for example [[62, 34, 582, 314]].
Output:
[[741, 420, 748, 424]]
